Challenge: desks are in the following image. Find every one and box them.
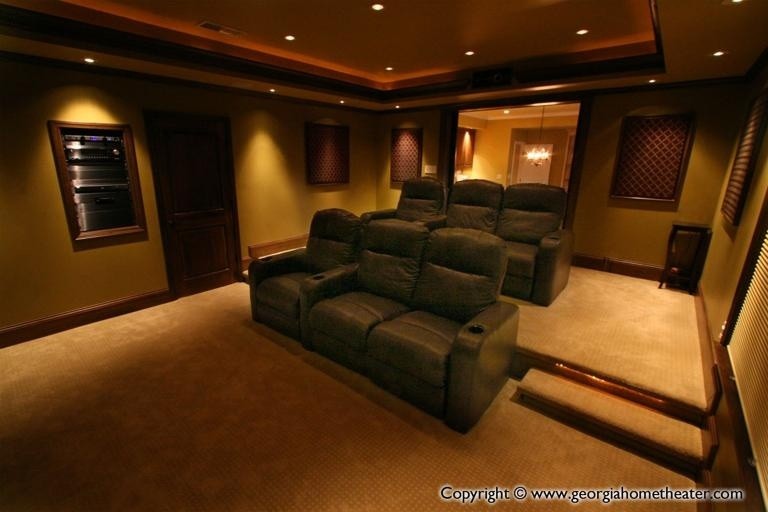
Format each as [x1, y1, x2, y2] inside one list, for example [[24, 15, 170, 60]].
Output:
[[658, 220, 711, 296]]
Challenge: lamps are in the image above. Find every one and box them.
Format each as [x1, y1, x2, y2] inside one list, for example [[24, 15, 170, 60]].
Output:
[[526, 106, 552, 168]]
